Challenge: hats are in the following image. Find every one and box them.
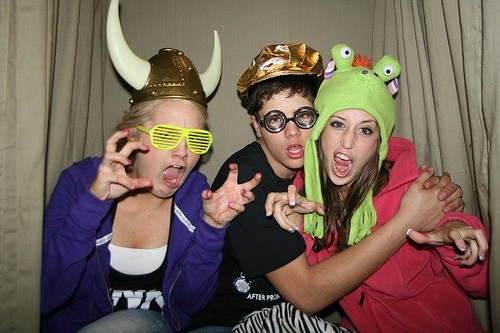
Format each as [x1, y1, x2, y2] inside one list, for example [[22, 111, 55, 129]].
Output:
[[303, 43, 401, 246], [236, 43, 325, 92]]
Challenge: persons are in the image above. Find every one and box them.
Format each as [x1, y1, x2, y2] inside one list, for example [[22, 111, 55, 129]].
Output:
[[209, 44, 466, 333], [265, 43, 490, 333], [40, 47, 261, 333]]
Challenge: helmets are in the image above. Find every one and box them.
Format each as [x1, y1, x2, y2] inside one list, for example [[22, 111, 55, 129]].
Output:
[[106, 0, 221, 109]]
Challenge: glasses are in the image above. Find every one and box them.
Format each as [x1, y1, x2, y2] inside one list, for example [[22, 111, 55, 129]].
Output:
[[136, 123, 213, 154], [258, 106, 319, 133]]
